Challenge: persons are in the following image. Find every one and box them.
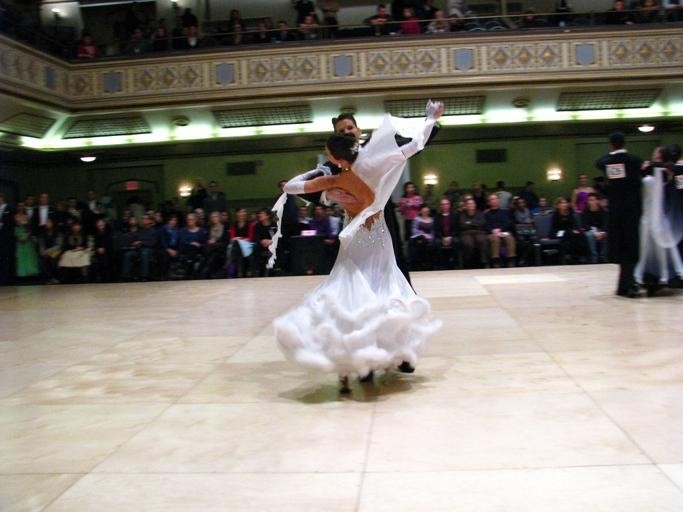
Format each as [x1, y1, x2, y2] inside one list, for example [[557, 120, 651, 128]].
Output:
[[272, 99, 442, 395], [2, 131, 683, 287], [294, 99, 445, 374], [74, 2, 682, 60], [597, 134, 682, 298]]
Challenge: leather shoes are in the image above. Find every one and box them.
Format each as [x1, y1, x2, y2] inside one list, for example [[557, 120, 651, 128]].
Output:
[[626, 277, 683, 298], [340, 361, 414, 394]]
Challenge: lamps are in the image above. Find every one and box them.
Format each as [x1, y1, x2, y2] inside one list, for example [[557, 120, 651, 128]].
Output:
[[177, 181, 193, 197], [637, 123, 656, 133], [422, 172, 438, 196], [79, 156, 96, 163], [545, 167, 562, 185]]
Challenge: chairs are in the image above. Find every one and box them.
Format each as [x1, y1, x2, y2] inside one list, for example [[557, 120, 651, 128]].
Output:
[[0, 193, 609, 279]]
